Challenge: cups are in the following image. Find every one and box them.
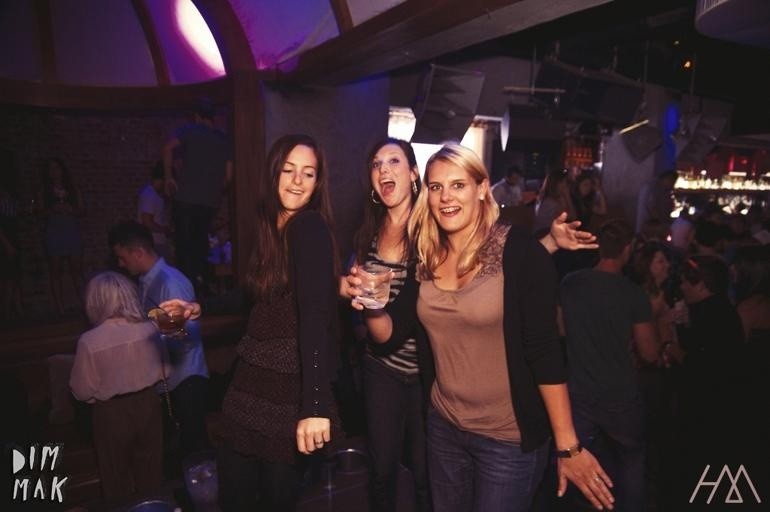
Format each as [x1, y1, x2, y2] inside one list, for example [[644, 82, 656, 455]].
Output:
[[187, 457, 217, 504], [159, 307, 184, 340], [358, 266, 390, 309]]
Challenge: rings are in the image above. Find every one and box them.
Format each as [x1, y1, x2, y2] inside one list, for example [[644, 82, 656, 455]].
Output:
[[315, 441, 324, 445]]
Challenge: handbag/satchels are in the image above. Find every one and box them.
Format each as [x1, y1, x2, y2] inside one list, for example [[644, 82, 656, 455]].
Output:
[[162, 428, 194, 466]]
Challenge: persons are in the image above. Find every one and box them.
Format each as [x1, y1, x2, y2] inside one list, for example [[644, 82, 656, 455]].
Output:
[[105, 220, 213, 482], [135, 157, 177, 260], [489, 155, 770, 511], [0, 155, 39, 323], [66, 271, 172, 512], [36, 151, 94, 322], [156, 132, 343, 511], [163, 96, 236, 318]]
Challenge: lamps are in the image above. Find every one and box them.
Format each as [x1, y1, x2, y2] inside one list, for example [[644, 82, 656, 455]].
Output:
[[500, 28, 569, 156]]
